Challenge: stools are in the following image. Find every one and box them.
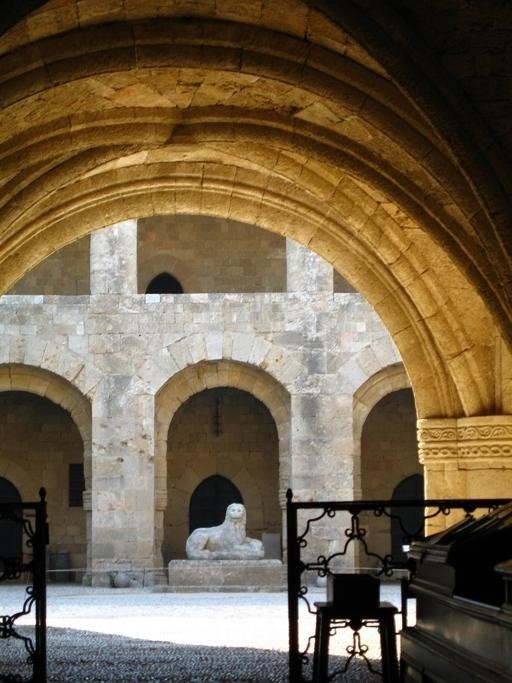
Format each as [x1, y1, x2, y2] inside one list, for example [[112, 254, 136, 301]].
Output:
[[311, 600, 399, 682]]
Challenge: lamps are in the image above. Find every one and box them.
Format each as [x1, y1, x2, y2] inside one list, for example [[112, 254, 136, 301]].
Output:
[[400, 535, 411, 553]]
